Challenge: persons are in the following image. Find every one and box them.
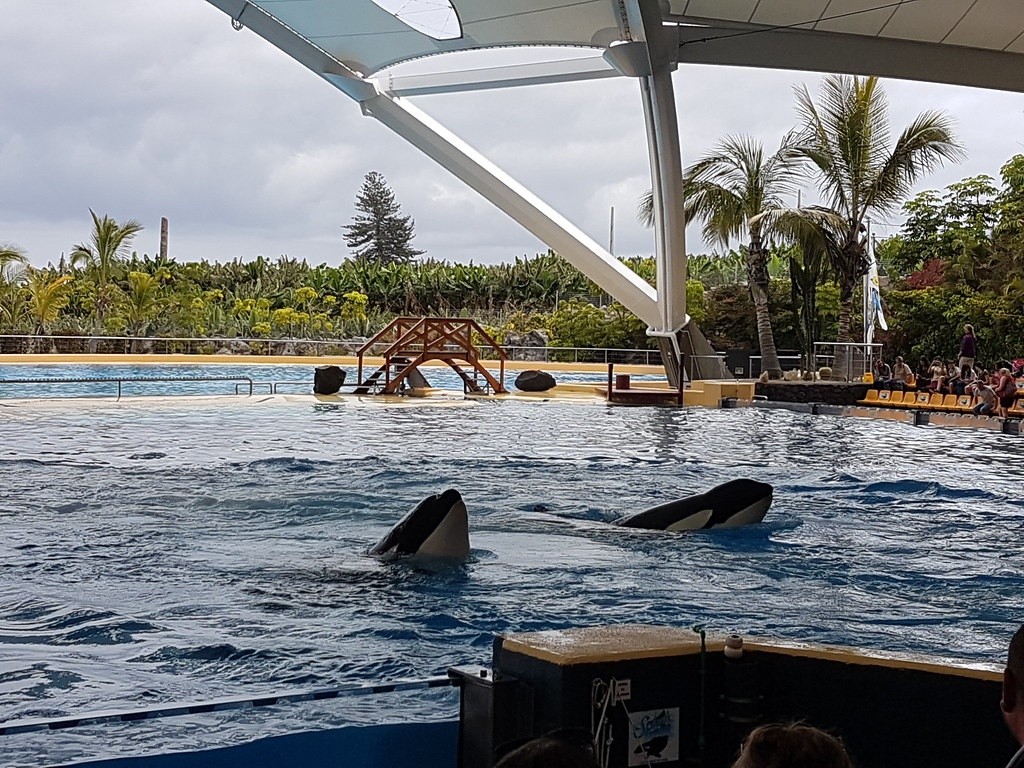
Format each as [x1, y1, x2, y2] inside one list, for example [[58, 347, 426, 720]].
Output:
[[995, 367, 1017, 418], [957, 324, 976, 370], [972, 380, 998, 417], [873, 356, 990, 396], [736, 725, 851, 767], [998, 625, 1024, 768]]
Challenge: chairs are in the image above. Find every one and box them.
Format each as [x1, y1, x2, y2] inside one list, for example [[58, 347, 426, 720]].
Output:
[[863, 372, 1024, 415]]
